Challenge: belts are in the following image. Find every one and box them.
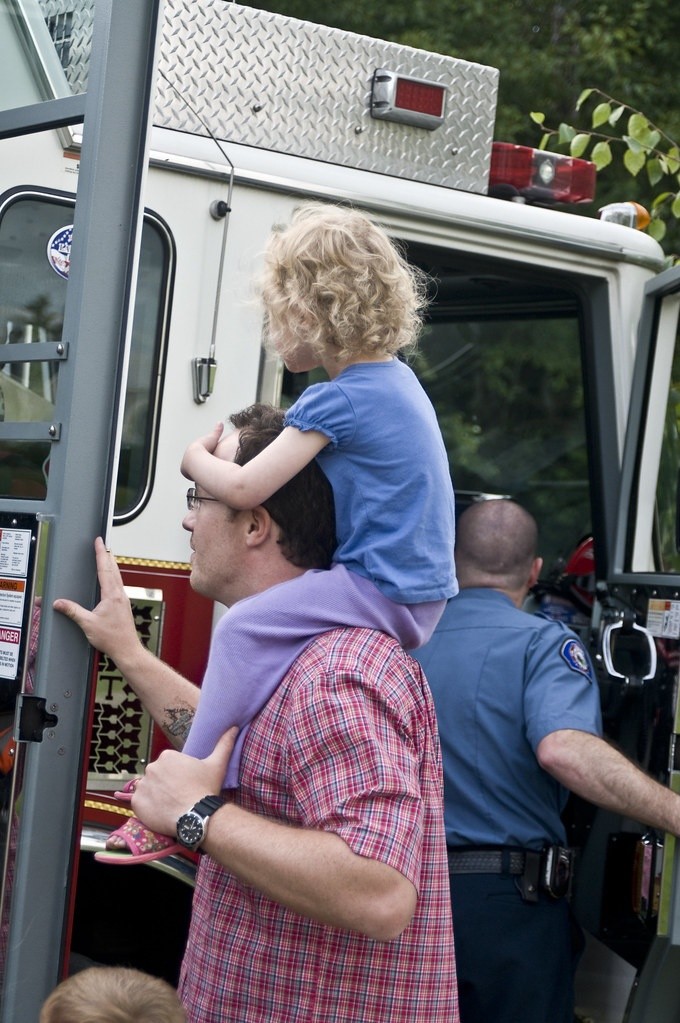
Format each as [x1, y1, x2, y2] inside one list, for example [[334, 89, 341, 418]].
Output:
[[448, 851, 525, 873]]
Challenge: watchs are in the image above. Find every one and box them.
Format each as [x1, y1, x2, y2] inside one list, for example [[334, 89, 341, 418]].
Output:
[[175, 795, 227, 855]]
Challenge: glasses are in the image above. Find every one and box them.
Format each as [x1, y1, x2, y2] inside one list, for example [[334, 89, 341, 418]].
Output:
[[187, 488, 219, 510]]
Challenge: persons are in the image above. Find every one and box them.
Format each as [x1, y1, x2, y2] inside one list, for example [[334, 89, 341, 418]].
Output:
[[37, 967, 186, 1023], [404, 499, 680, 1023], [51, 402, 460, 1023], [94, 205, 459, 864]]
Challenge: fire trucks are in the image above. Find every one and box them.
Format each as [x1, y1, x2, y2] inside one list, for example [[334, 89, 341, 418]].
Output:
[[0, 0, 680, 1023]]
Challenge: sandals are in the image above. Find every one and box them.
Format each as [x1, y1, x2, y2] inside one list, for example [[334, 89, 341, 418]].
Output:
[[94, 817, 187, 864], [114, 778, 142, 801]]
